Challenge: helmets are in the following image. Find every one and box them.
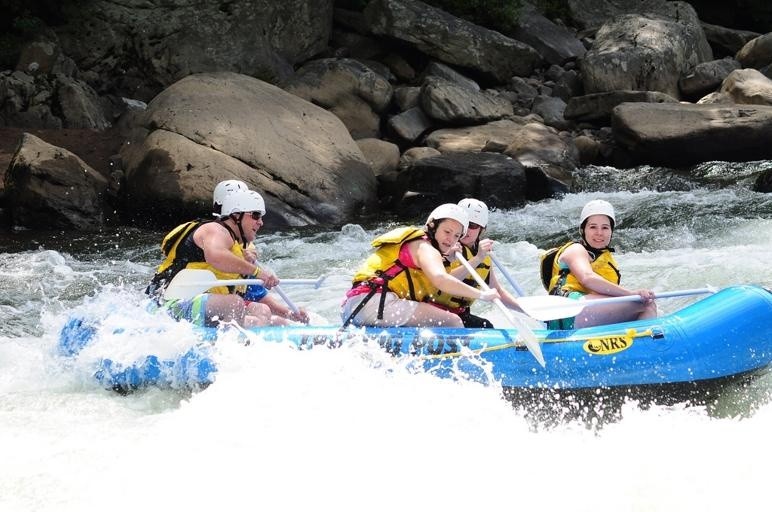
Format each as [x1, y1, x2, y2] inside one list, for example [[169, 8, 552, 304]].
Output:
[[578, 200, 616, 236], [424, 198, 489, 240], [213, 180, 266, 216]]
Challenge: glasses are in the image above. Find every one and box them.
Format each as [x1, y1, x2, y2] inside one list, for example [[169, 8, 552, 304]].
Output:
[[245, 212, 260, 220]]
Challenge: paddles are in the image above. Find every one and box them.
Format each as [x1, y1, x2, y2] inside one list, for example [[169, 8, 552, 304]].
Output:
[[454, 251, 545, 367], [164, 269, 325, 300], [515, 284, 718, 321]]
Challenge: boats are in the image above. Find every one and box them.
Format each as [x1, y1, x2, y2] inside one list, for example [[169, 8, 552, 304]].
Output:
[[52, 280, 772, 406]]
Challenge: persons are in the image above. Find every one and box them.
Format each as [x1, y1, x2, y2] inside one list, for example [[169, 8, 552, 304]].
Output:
[[147, 189, 280, 327], [339, 204, 500, 330], [212, 179, 309, 326], [544, 199, 659, 330], [422, 197, 527, 329]]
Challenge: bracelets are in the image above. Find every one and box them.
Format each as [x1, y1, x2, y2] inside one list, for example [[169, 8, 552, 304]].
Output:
[[252, 267, 261, 279]]
